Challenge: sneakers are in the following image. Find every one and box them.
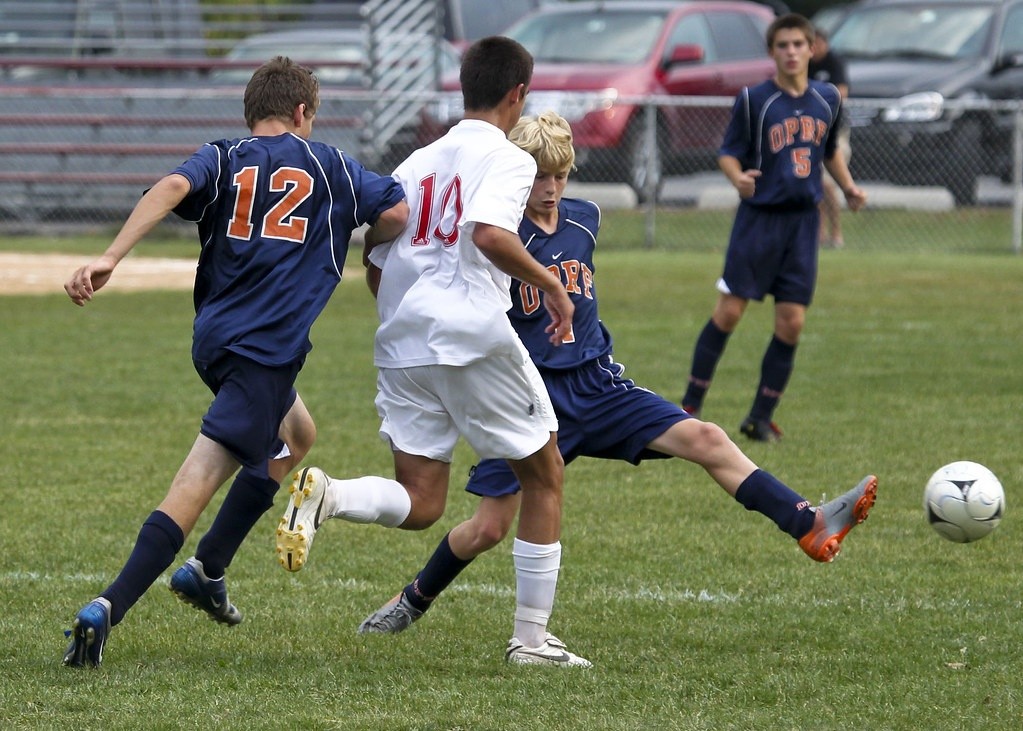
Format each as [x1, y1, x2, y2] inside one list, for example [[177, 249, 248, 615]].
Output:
[[276, 467, 334, 571], [739, 416, 781, 444], [357, 589, 424, 634], [506, 632, 594, 672], [167, 557, 241, 627], [61, 596, 112, 669]]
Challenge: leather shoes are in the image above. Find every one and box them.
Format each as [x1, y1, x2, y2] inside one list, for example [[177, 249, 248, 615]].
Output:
[[799, 474, 880, 562]]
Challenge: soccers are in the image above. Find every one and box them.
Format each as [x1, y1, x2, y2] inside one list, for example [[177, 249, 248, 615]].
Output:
[[923, 459, 1006, 543]]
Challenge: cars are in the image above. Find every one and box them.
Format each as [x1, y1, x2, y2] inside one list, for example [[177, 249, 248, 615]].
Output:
[[422, 2, 793, 196], [217, 30, 466, 89], [810, 0, 1023, 208]]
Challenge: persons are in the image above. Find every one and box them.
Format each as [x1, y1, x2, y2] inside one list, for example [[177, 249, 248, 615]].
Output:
[[677, 14, 867, 446], [62, 55, 408, 668], [359, 110, 878, 638], [809, 25, 853, 252], [279, 37, 594, 672]]
